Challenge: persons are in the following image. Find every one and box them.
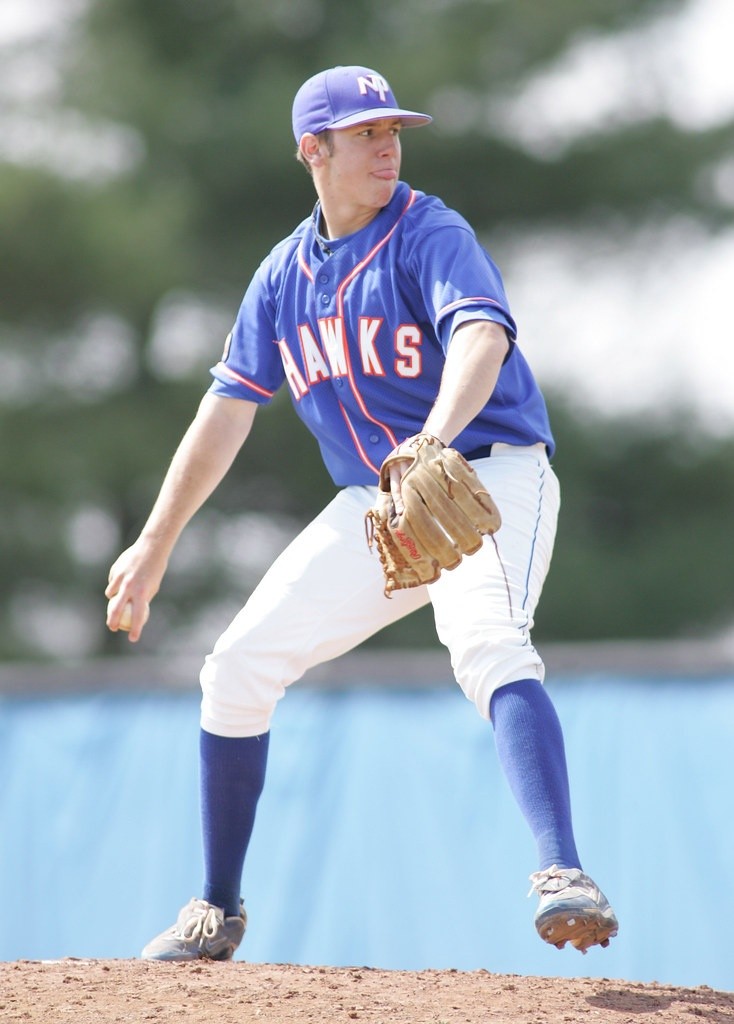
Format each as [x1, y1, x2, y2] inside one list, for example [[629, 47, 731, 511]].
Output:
[[105, 65, 618, 961]]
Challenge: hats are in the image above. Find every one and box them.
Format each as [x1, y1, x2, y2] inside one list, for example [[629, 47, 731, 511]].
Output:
[[291, 66, 433, 148]]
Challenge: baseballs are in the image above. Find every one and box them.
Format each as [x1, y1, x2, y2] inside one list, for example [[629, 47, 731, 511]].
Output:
[[106, 595, 150, 632]]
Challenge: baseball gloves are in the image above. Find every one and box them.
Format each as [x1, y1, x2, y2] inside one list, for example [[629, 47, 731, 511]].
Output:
[[367, 432, 503, 590]]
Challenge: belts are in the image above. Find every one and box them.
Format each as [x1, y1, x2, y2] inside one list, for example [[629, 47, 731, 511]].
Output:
[[460, 444, 491, 463]]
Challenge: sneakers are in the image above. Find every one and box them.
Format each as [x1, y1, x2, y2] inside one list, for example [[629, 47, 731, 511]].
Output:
[[526, 865, 618, 953], [140, 898, 249, 963]]
